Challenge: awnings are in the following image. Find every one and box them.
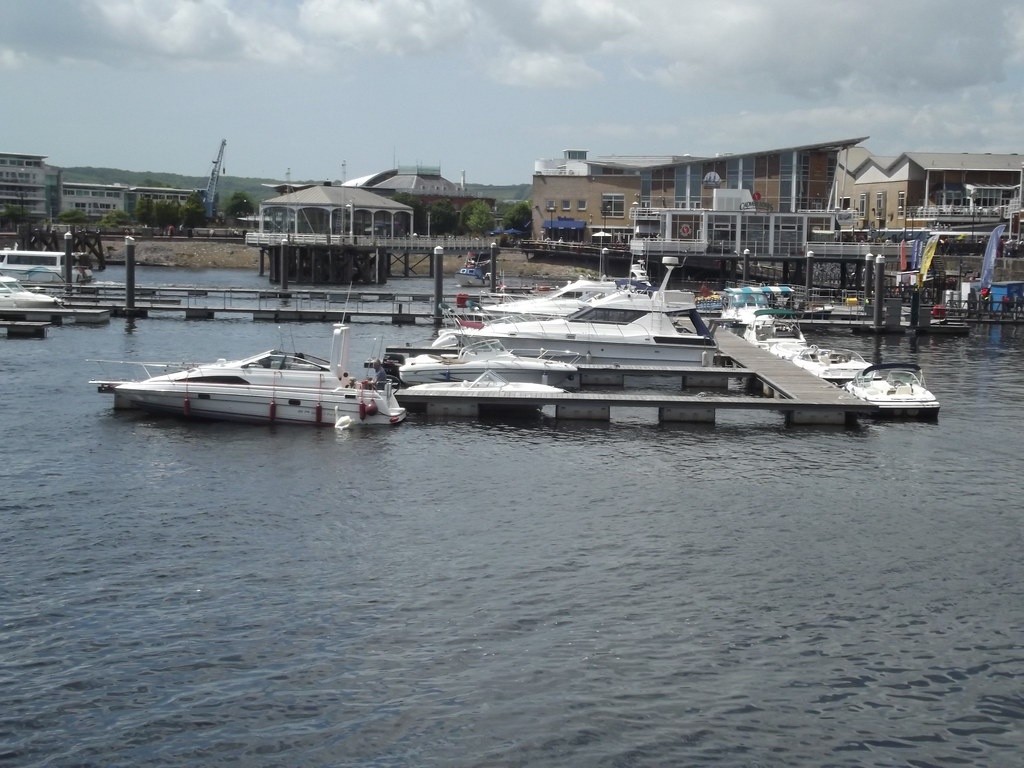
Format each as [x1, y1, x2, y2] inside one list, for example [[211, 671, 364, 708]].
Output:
[[542, 220, 587, 229]]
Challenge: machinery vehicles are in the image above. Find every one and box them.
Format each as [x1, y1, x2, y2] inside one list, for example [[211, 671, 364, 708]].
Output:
[[201, 137, 228, 227]]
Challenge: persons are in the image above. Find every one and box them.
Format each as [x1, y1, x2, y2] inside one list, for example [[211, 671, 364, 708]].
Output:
[[558, 236, 564, 249], [544, 235, 552, 250], [475, 236, 480, 239], [649, 232, 663, 240], [836, 234, 1024, 258], [445, 232, 457, 240], [405, 230, 418, 240]]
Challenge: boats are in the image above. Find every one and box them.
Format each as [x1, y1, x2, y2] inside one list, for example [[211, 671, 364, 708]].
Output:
[[111, 323, 409, 431], [718, 282, 835, 323], [0, 275, 64, 310], [0, 249, 93, 284], [770, 340, 807, 360], [742, 309, 810, 348], [391, 246, 709, 386], [842, 360, 940, 419], [791, 344, 883, 383]]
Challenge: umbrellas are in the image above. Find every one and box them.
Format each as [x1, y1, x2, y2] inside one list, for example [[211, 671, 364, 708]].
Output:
[[490, 228, 523, 236]]
